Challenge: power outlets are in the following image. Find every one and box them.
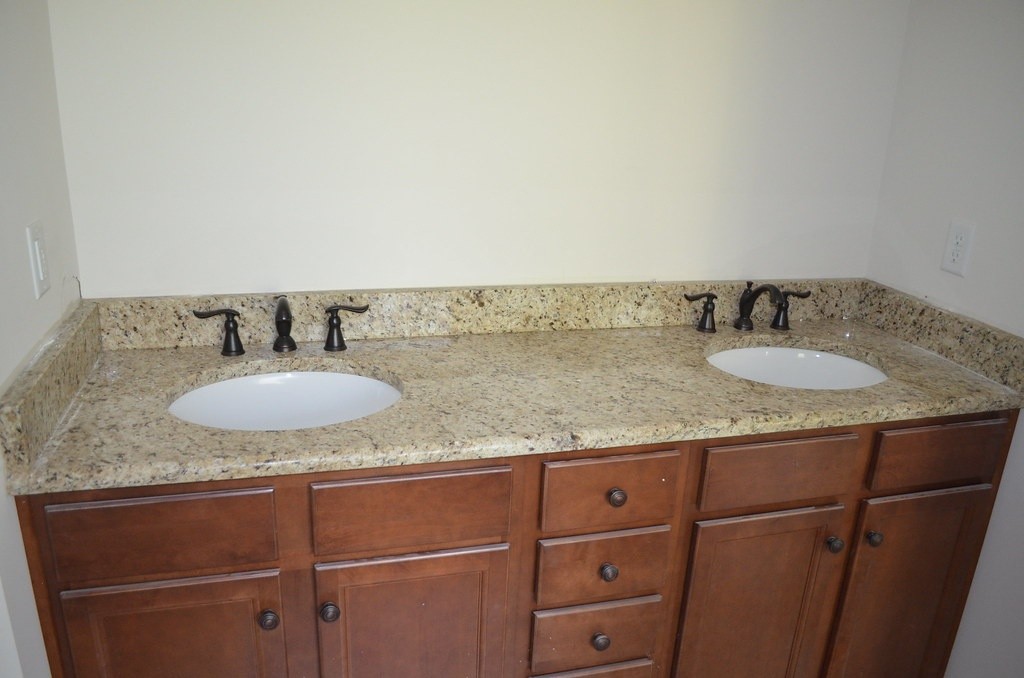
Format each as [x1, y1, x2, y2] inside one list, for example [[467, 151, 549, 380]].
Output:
[[26, 218, 52, 299], [940, 220, 977, 277]]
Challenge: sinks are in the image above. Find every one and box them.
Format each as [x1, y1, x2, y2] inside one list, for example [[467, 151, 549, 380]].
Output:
[[157, 350, 406, 433], [698, 325, 895, 399]]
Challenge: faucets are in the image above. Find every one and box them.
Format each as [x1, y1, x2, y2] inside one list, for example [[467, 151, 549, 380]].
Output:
[[273, 296, 297, 352], [733, 280, 784, 332]]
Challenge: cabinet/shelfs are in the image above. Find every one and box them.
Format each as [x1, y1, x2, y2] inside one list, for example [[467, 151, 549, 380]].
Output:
[[15, 408, 1024, 678]]
